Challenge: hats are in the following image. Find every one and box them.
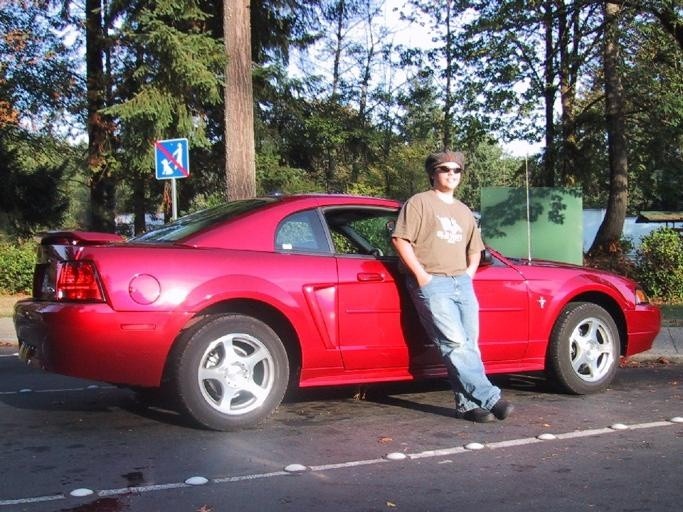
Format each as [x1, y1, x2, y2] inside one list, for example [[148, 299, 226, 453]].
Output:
[[425, 150, 465, 172]]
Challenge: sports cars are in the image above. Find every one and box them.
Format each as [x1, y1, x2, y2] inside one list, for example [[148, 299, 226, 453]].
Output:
[[11, 190, 663, 433]]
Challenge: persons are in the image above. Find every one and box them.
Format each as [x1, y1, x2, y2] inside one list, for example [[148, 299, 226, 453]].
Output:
[[388, 149, 515, 425]]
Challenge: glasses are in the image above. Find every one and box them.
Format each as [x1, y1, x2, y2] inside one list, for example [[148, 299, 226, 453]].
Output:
[[432, 166, 463, 174]]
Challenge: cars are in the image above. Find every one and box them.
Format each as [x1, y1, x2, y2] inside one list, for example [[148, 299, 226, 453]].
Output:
[[112, 208, 169, 235]]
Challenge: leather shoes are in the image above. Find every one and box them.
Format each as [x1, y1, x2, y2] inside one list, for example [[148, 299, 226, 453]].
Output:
[[489, 398, 514, 422], [455, 406, 495, 424]]
